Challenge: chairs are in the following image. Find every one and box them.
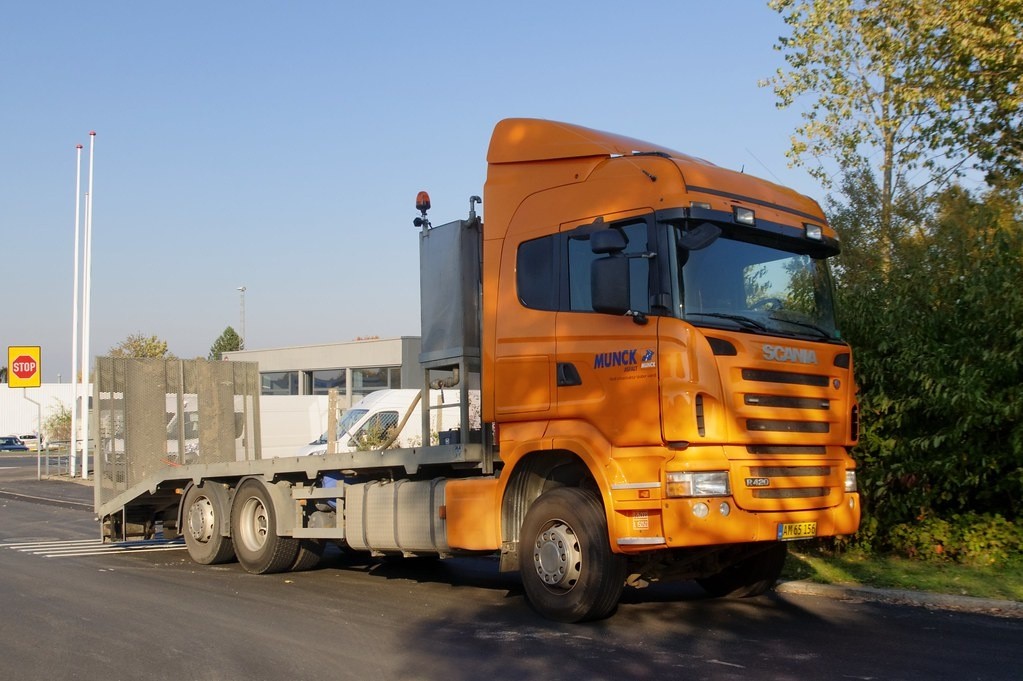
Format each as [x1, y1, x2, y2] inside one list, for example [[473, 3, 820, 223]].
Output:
[[6, 441, 13, 445]]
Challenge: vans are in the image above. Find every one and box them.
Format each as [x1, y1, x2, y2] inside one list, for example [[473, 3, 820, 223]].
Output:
[[102, 425, 126, 482], [294, 389, 495, 456], [166, 395, 340, 466]]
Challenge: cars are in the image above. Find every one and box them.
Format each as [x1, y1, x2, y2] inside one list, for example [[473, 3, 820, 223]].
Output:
[[17, 434, 38, 450], [0, 435, 29, 452]]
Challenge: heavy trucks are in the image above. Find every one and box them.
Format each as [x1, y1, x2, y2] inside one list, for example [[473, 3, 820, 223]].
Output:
[[90, 119, 861, 620]]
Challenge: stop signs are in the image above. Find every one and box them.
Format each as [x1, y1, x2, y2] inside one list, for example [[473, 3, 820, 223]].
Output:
[[7, 345, 42, 388]]
